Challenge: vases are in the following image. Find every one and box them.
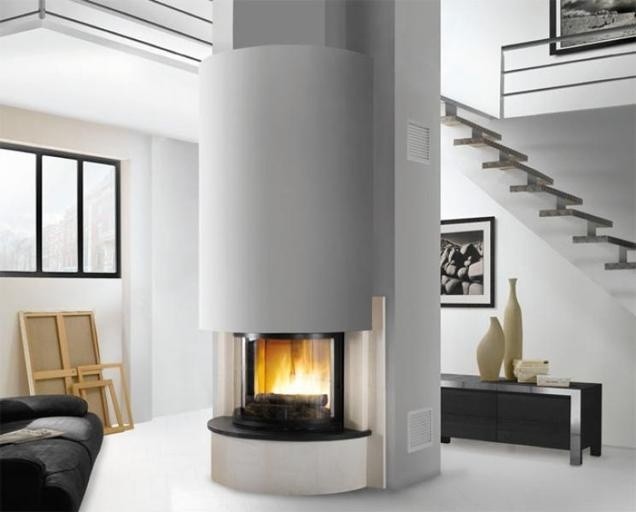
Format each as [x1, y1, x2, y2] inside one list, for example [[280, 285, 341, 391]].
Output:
[[476, 316, 505, 381]]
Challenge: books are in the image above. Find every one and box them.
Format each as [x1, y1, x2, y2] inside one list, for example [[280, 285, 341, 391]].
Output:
[[0, 427, 68, 446], [512, 359, 570, 387]]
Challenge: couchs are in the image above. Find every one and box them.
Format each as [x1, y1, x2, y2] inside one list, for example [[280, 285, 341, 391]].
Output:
[[0, 395, 103, 512]]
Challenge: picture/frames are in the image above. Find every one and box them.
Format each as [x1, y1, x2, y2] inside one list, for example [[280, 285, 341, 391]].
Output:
[[550, 0, 636, 55], [440, 216, 495, 308]]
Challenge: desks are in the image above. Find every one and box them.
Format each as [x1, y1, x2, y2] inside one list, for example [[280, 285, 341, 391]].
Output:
[[441, 374, 602, 466]]
